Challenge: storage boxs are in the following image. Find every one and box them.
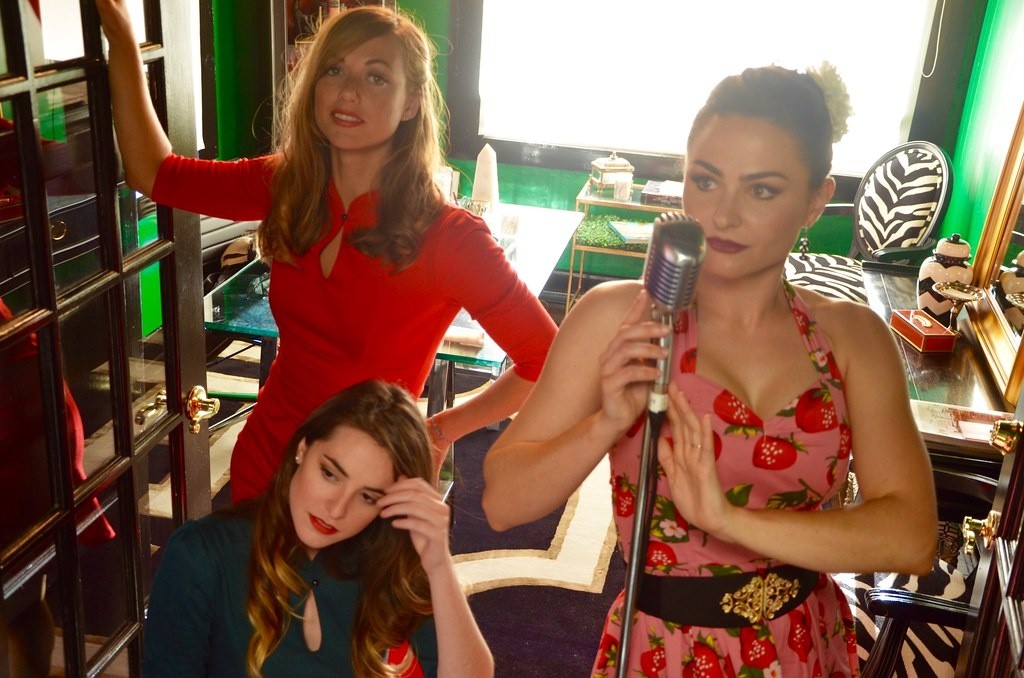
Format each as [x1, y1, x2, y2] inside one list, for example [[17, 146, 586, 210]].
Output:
[[889, 309, 955, 353]]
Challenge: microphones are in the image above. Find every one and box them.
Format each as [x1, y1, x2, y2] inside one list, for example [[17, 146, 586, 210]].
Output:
[[642, 210, 707, 443]]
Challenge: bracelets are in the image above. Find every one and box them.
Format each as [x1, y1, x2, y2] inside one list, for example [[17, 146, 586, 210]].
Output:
[[432, 413, 452, 443]]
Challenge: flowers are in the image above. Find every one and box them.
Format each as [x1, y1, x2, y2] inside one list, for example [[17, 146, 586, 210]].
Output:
[[806, 60, 854, 143]]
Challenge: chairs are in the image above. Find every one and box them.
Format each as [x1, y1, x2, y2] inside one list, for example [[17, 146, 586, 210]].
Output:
[[784, 141, 953, 304], [834, 467, 1024, 678]]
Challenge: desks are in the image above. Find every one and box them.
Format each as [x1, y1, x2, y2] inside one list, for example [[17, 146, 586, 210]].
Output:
[[204, 197, 587, 416], [863, 267, 1006, 458]]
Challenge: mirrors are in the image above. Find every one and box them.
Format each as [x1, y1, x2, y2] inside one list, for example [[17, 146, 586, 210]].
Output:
[[963, 103, 1024, 412]]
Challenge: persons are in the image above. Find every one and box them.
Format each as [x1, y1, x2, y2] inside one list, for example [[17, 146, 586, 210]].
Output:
[[481, 63, 938, 678], [147, 381, 495, 678], [96, 0, 558, 506]]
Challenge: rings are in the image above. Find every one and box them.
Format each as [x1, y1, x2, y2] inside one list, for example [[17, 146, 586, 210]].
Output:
[[691, 444, 701, 448]]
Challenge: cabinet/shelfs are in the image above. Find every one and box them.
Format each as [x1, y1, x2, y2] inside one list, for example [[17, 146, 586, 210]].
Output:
[[0, 0, 212, 678], [271, 0, 396, 109], [566, 180, 683, 316]]
